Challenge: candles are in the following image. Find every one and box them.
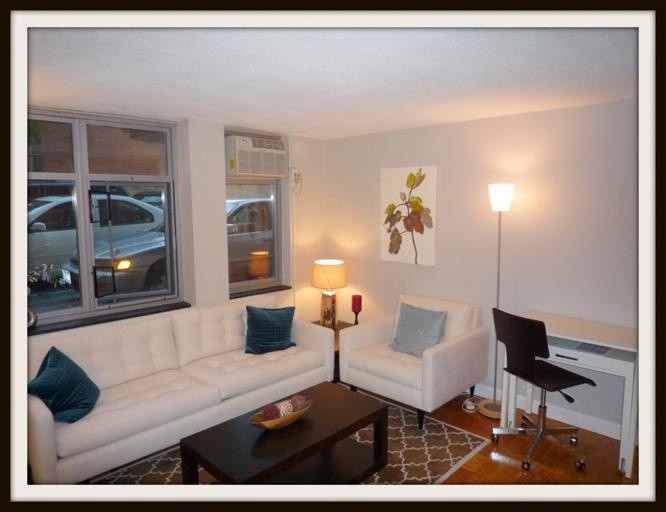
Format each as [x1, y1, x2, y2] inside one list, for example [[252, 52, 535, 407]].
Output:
[[351, 294, 362, 312]]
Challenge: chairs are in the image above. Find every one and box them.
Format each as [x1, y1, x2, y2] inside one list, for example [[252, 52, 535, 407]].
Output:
[[337, 291, 492, 428], [491, 305, 598, 473]]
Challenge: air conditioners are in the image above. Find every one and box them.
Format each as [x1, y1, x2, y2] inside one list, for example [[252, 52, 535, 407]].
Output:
[[225, 135, 290, 185]]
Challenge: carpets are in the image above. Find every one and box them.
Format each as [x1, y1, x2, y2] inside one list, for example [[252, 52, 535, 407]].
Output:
[[90, 382, 491, 483]]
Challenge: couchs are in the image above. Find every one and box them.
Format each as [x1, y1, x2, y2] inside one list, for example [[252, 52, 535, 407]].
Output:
[[26, 287, 340, 484]]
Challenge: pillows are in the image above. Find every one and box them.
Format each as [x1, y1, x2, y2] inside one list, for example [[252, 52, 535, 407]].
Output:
[[243, 305, 297, 354], [27, 346, 101, 424], [389, 303, 449, 358]]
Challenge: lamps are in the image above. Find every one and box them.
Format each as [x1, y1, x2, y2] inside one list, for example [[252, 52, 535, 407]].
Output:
[[247, 250, 272, 279], [310, 258, 348, 328], [478, 184, 516, 420]]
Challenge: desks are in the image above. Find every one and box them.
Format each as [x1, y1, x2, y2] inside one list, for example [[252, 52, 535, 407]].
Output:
[[498, 308, 639, 479], [313, 320, 353, 384]]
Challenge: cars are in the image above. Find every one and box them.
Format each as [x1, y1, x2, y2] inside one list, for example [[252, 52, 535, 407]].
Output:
[[53, 196, 274, 301], [135, 195, 161, 206], [26, 192, 167, 287]]
